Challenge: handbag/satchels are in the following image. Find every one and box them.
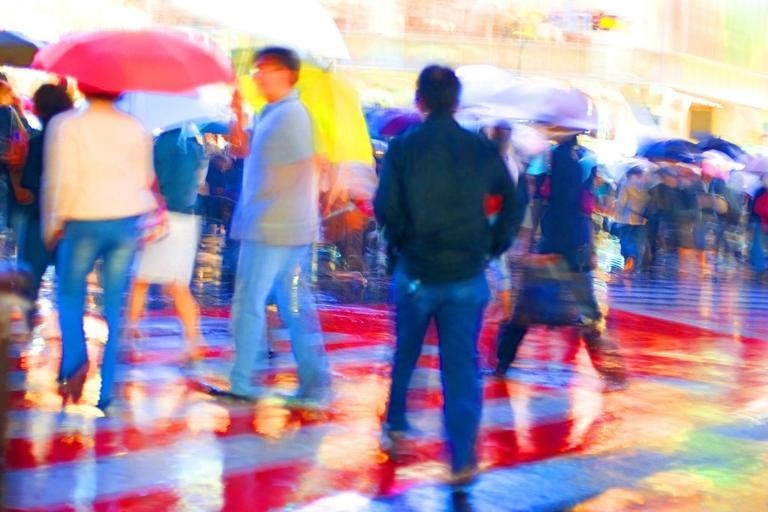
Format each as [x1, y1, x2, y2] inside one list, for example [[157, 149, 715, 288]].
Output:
[[508, 262, 603, 330]]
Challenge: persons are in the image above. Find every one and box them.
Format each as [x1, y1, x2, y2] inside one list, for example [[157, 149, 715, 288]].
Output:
[[2, 33, 768, 478]]
